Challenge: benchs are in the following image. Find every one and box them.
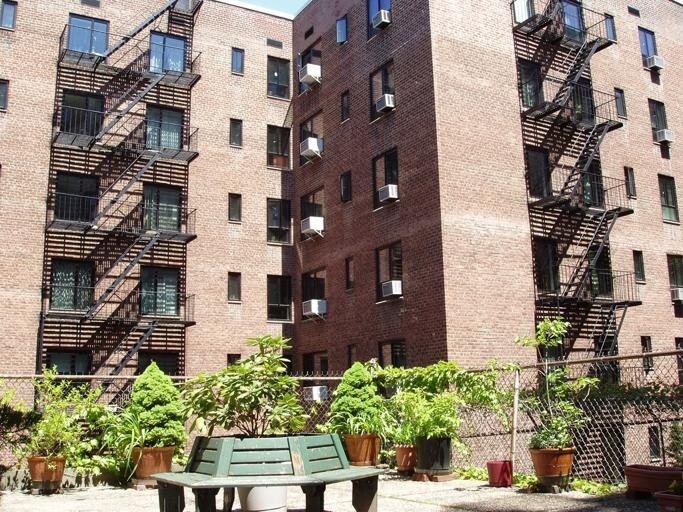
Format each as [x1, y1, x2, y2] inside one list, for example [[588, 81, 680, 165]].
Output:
[[148, 429, 388, 512]]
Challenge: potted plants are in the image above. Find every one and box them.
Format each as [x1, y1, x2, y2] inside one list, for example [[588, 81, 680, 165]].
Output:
[[183, 332, 312, 511], [9, 360, 184, 494]]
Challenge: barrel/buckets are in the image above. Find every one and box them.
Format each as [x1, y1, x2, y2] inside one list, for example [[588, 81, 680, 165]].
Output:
[[413, 436, 452, 475], [236, 485, 288, 512], [486, 459, 513, 488]]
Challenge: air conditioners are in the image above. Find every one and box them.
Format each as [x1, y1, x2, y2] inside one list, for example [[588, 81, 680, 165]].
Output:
[[669, 288, 683, 301], [370, 8, 403, 300], [646, 53, 675, 145], [296, 62, 328, 404]]
